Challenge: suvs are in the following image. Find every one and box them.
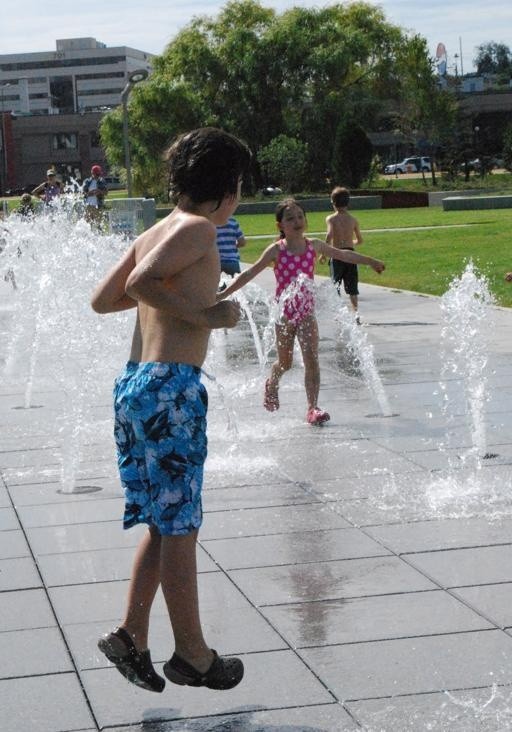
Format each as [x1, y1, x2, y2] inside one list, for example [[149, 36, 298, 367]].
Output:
[[385, 156, 437, 174]]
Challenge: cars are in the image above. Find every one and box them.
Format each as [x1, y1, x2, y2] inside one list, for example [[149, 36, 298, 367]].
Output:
[[460, 156, 505, 172], [104, 176, 124, 190]]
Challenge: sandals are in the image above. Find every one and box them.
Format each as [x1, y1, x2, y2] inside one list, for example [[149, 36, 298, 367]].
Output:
[[263, 378, 280, 414], [163, 645, 245, 691], [306, 406, 332, 426], [98, 627, 166, 693]]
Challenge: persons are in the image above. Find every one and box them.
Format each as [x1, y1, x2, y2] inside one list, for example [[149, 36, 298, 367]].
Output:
[[31, 169, 64, 208], [216, 198, 385, 426], [82, 165, 108, 231], [91, 128, 253, 693], [319, 187, 363, 325], [11, 193, 35, 223], [216, 217, 247, 293]]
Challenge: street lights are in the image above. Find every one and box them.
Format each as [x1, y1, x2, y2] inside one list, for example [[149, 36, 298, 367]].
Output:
[[474, 126, 481, 148], [0, 83, 10, 190], [119, 67, 148, 198]]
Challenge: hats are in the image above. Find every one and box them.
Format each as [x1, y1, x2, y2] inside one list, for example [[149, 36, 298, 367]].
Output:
[[91, 166, 102, 176], [47, 169, 56, 177]]
